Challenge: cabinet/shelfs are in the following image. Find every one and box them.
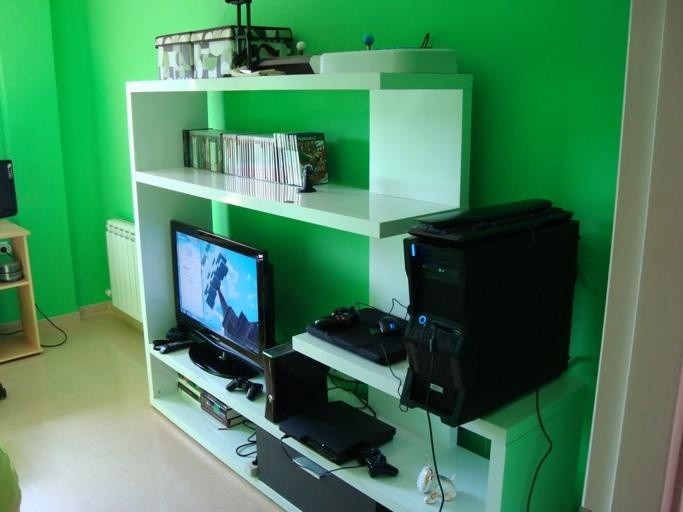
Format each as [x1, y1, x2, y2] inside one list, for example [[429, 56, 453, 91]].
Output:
[[0, 220, 44, 364], [125, 69, 588, 511]]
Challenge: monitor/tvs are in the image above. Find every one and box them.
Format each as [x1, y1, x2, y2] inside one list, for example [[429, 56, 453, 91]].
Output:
[[1, 160, 20, 222], [168, 218, 274, 380]]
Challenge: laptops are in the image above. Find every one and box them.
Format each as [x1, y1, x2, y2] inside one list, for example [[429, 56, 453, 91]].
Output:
[[277, 398, 397, 465], [312, 307, 409, 366]]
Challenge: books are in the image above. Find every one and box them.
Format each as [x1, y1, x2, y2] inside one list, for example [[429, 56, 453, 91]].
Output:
[[176, 372, 247, 428], [181, 127, 329, 186], [290, 454, 327, 479], [222, 173, 303, 208]]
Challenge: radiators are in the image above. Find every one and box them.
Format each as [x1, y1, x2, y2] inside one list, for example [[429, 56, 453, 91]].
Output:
[[102, 217, 142, 321]]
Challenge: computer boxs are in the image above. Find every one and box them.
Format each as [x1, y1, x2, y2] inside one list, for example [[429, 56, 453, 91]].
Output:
[[397, 197, 579, 429]]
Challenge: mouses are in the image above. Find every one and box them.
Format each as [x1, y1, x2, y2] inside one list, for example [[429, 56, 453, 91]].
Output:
[[378, 313, 401, 338]]
